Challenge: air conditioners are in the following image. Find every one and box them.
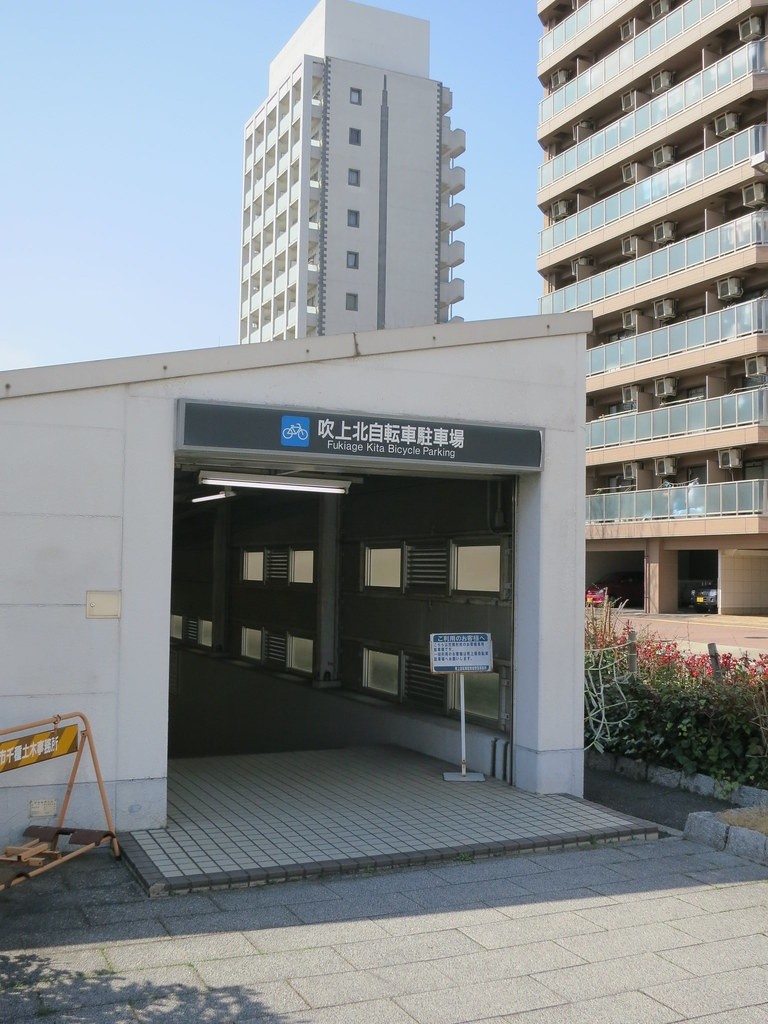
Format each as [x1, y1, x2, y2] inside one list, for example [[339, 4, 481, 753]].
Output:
[[622, 309, 642, 330], [739, 16, 762, 43], [622, 385, 642, 404], [657, 457, 677, 474], [655, 377, 676, 397], [622, 235, 644, 258], [654, 222, 676, 244], [624, 462, 642, 478], [718, 278, 743, 301], [742, 183, 768, 208], [551, 0, 675, 221], [654, 299, 677, 321], [571, 257, 590, 277], [746, 356, 767, 378], [715, 113, 740, 139], [718, 448, 742, 469]]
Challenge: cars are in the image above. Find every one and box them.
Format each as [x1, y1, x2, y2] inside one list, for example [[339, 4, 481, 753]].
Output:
[[690, 575, 718, 614], [585, 570, 644, 608]]
